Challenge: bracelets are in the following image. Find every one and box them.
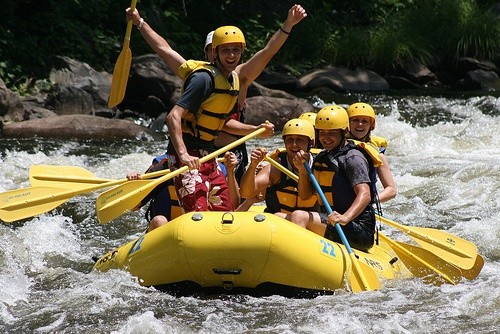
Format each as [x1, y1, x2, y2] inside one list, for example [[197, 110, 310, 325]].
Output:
[[280, 26, 290, 34], [137, 18, 143, 29]]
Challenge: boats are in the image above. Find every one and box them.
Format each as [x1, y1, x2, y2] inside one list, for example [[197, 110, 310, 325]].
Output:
[[90, 205, 414, 300]]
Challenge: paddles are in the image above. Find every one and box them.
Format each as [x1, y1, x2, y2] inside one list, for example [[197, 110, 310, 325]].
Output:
[[108, 0, 136, 109], [29, 164, 117, 189], [96, 128, 265, 224], [0, 169, 170, 222], [264, 155, 484, 286], [302, 158, 382, 295]]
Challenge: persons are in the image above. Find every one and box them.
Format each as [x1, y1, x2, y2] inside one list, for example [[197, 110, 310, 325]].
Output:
[[166, 26, 275, 212], [240, 103, 397, 251], [126, 4, 306, 197], [124, 146, 239, 233]]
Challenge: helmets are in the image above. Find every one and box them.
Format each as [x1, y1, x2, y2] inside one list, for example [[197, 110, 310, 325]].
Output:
[[282, 118, 315, 146], [315, 106, 350, 132], [298, 113, 317, 127], [347, 103, 376, 131], [204, 30, 215, 59], [212, 26, 246, 53]]
